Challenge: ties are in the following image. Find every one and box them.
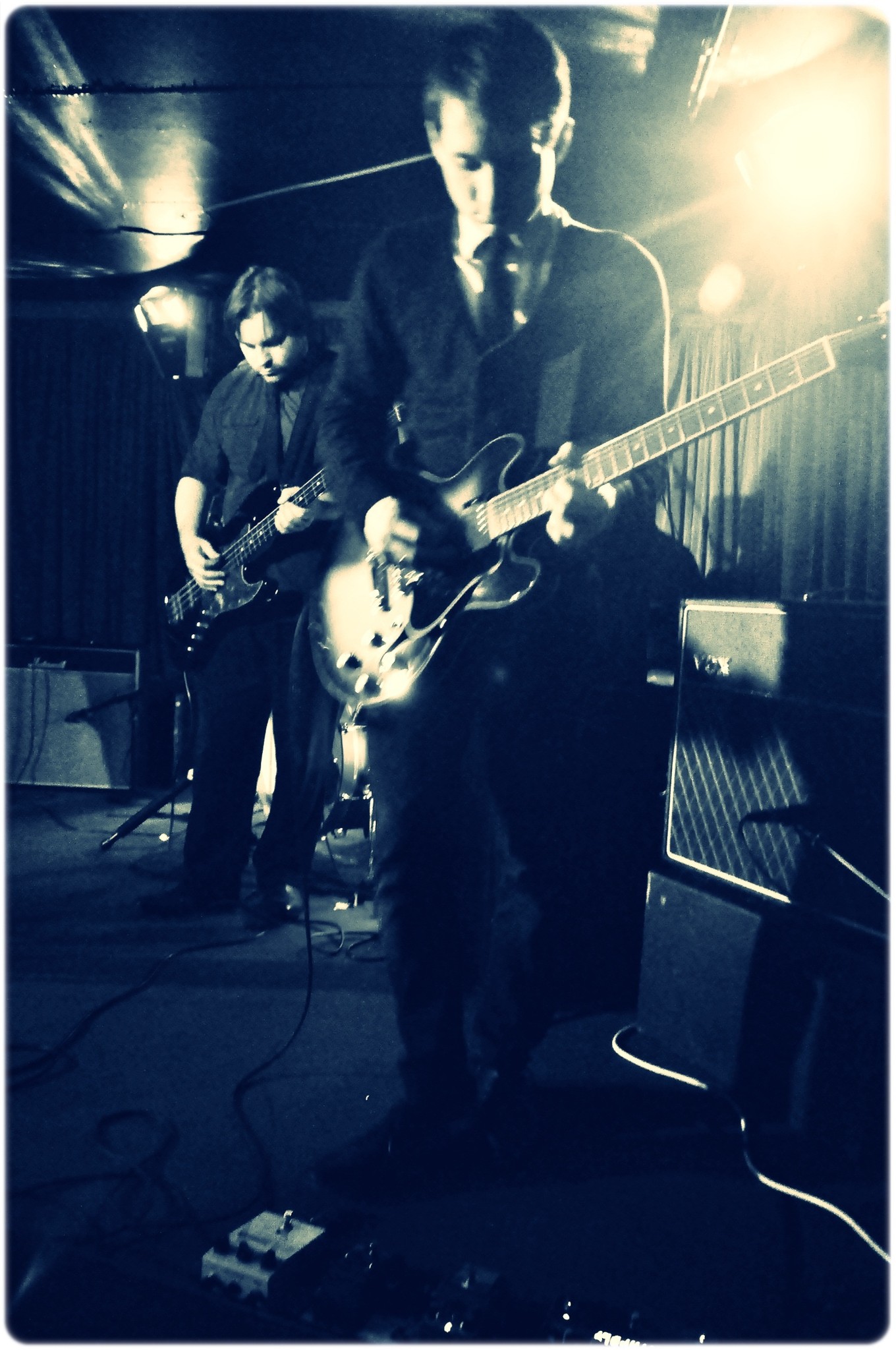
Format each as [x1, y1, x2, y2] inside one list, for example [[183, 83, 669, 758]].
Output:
[[475, 238, 514, 351]]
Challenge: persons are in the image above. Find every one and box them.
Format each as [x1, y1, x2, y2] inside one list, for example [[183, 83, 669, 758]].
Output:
[[141, 263, 342, 918], [319, 4, 670, 1341]]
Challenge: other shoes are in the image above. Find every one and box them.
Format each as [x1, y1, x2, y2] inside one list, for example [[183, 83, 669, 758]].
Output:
[[317, 1104, 493, 1199], [253, 880, 306, 918], [137, 883, 240, 915]]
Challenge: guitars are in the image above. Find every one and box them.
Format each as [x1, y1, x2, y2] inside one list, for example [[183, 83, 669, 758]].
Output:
[[164, 466, 329, 654], [309, 295, 891, 709]]
